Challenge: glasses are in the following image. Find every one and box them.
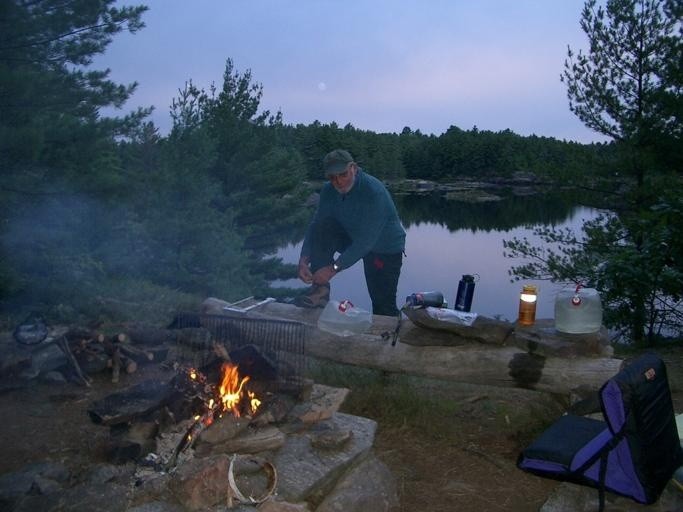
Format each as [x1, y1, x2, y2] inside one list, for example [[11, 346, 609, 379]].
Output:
[[329, 171, 347, 181]]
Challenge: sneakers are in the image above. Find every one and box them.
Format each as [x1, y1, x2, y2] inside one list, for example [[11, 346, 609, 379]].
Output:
[[299, 282, 330, 308]]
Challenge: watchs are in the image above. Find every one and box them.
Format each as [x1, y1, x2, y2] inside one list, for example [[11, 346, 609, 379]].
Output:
[[332, 262, 341, 274]]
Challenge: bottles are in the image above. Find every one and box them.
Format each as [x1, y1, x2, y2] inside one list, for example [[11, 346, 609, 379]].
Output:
[[518, 284, 538, 326], [405, 290, 444, 307], [454, 273, 480, 313]]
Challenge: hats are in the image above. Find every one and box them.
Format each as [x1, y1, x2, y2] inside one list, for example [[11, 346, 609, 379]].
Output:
[[323, 149, 353, 176]]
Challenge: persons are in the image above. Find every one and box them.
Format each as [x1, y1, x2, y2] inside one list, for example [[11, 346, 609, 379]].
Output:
[[293, 149, 406, 316]]
